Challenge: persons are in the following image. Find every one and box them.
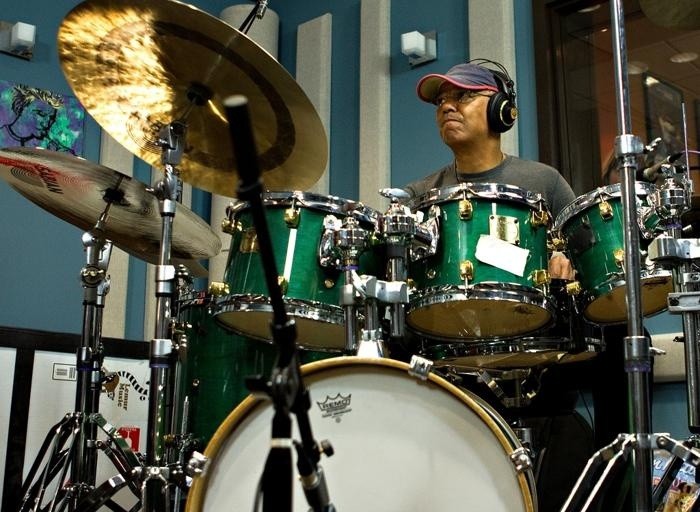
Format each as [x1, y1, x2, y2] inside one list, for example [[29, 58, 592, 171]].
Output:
[[372, 56, 656, 512], [601, 147, 645, 187]]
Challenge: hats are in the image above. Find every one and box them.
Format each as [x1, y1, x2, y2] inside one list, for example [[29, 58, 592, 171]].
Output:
[[417, 63, 499, 103]]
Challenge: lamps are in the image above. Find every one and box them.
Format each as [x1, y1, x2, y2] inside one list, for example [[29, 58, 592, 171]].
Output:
[[0, 21, 36, 60], [401, 30, 437, 68]]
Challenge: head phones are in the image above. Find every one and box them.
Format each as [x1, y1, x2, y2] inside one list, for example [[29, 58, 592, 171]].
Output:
[[467, 58, 519, 134]]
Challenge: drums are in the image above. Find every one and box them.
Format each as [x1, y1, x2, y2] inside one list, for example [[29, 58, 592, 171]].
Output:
[[391, 289, 602, 378], [555, 180, 672, 327], [210, 191, 383, 353], [169, 286, 359, 447], [183, 354, 538, 511], [405, 182, 557, 341]]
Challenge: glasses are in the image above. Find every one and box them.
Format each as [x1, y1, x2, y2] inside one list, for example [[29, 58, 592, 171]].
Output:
[[435, 89, 492, 103]]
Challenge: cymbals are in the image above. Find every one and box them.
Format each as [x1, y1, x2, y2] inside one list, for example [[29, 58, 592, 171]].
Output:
[[58, 1, 328, 201], [0, 146, 226, 265]]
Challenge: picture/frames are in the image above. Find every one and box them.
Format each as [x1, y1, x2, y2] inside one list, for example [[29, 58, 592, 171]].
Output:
[[641, 72, 684, 167]]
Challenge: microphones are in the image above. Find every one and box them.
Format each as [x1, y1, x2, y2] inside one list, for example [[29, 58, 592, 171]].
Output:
[[636, 152, 683, 184]]
